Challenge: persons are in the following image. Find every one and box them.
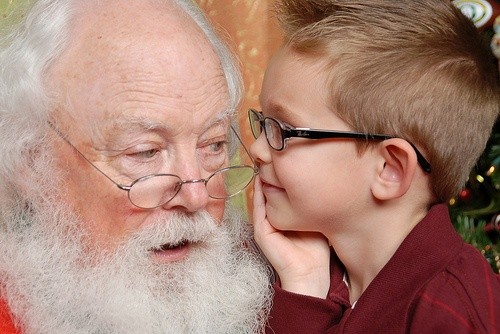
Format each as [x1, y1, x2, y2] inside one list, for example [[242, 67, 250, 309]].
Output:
[[0, 0, 275, 334], [248, 0, 500, 334]]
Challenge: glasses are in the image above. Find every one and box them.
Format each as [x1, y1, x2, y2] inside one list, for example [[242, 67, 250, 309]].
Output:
[[48, 120, 261, 209], [248, 109, 432, 174]]
